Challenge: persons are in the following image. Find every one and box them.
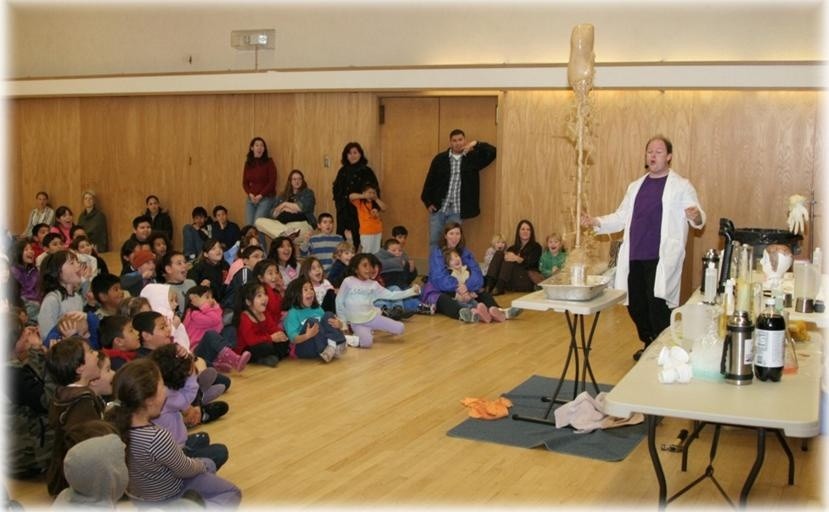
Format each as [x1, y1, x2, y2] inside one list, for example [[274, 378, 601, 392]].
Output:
[[271, 170, 318, 230], [417, 128, 496, 286], [330, 142, 382, 249], [423, 215, 567, 323], [348, 180, 387, 255], [237, 216, 435, 368], [581, 138, 709, 360], [240, 135, 278, 224], [8, 186, 243, 508]]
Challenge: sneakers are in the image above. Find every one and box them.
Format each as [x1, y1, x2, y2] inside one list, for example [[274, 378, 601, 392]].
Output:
[[320, 334, 360, 363], [458, 302, 523, 324], [380, 302, 437, 319], [202, 401, 228, 423], [286, 227, 300, 241], [258, 355, 278, 366], [197, 368, 226, 405]]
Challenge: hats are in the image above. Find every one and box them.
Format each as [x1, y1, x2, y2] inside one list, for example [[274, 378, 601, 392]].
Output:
[[134, 249, 157, 268]]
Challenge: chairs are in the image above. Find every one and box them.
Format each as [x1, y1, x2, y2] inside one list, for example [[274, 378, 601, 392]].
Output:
[[717, 218, 804, 293]]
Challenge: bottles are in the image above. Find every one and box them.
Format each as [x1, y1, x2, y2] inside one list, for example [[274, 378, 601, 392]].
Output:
[[783, 314, 800, 375], [720, 310, 755, 384], [717, 279, 735, 336], [753, 298, 786, 384], [701, 247, 719, 307]]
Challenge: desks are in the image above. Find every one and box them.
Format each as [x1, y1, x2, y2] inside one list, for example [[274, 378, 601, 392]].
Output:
[[686, 267, 823, 327], [511, 290, 627, 434], [607, 327, 824, 511]]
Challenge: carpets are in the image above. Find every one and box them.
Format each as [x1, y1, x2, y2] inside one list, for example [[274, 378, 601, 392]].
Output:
[[446, 375, 659, 461]]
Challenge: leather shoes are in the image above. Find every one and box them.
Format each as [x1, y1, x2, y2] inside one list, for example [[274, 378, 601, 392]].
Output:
[[478, 285, 504, 296]]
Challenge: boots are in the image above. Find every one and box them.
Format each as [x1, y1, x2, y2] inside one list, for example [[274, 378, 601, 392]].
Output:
[[212, 345, 251, 373]]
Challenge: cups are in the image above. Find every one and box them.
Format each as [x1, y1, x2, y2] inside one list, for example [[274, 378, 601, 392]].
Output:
[[791, 262, 822, 306], [669, 303, 714, 351]]
